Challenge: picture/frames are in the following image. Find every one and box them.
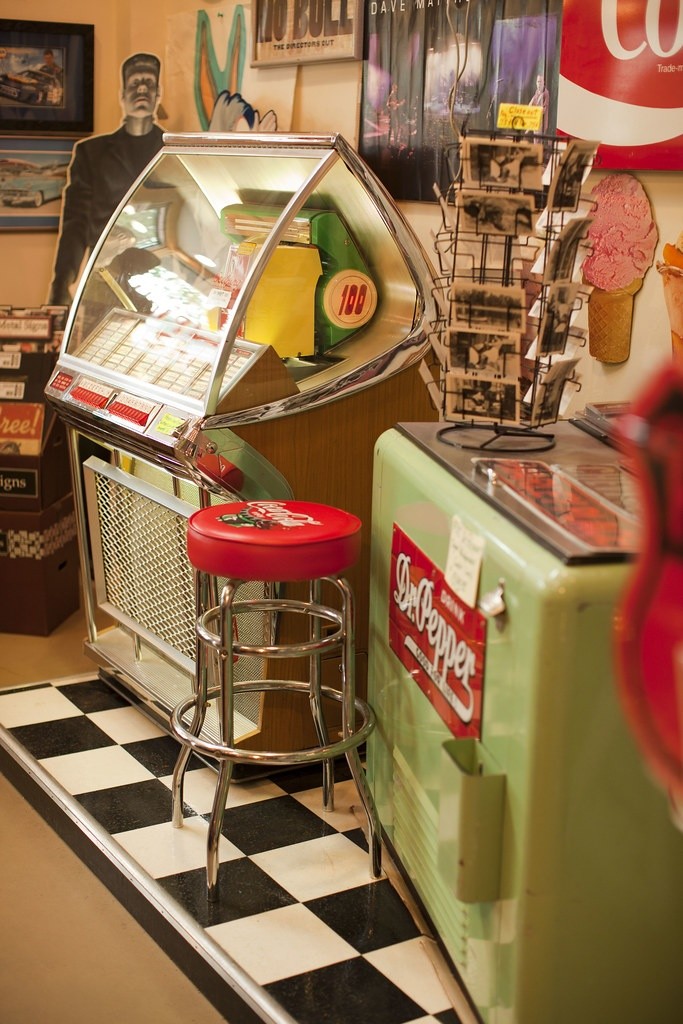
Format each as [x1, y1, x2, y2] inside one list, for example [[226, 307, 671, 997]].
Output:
[[0, 18, 95, 235], [248, 0, 365, 69]]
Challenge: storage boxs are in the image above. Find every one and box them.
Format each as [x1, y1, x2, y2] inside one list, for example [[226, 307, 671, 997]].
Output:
[[0, 492, 80, 637]]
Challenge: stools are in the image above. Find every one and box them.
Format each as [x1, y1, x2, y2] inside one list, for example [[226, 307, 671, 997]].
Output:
[[169, 500, 384, 903]]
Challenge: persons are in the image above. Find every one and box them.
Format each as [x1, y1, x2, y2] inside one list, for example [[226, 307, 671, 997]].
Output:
[[41, 49, 63, 83]]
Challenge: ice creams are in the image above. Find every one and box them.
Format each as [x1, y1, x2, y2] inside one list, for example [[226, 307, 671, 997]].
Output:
[[655, 230, 683, 365], [577, 173, 659, 364]]
